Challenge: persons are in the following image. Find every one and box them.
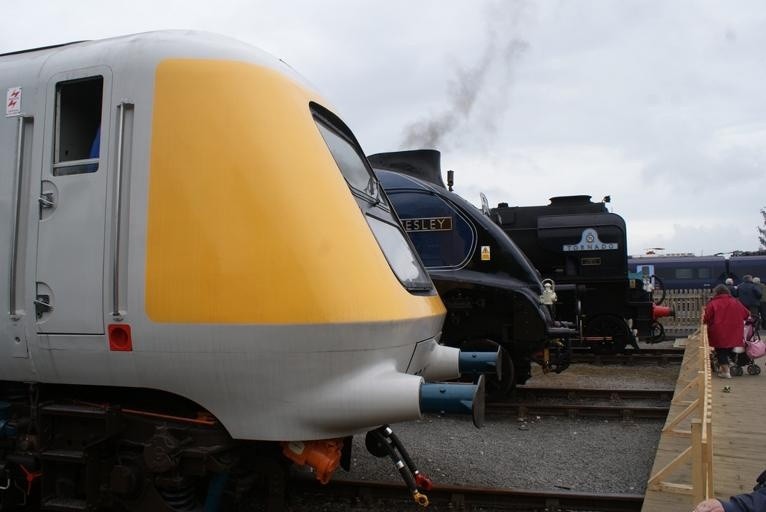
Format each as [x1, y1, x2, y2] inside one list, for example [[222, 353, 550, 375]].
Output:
[[704, 283, 751, 378], [723, 278, 739, 297], [751, 277, 765, 330], [739, 275, 762, 318], [693, 471, 765, 512]]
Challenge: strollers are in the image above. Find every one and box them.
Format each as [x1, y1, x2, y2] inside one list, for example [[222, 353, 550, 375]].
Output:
[[711, 312, 761, 376]]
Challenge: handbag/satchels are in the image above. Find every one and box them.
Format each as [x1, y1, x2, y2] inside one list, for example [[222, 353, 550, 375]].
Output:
[[745, 330, 766, 360]]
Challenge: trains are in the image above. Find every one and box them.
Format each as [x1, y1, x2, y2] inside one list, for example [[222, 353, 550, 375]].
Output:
[[366, 148, 584, 398], [0, 29, 502, 508], [627, 250, 765, 288], [478, 195, 675, 355]]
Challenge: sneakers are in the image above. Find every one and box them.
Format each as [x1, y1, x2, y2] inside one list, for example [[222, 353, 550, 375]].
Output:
[[718, 373, 731, 379]]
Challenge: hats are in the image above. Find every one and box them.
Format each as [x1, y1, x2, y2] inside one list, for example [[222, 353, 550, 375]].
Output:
[[725, 278, 733, 285]]
